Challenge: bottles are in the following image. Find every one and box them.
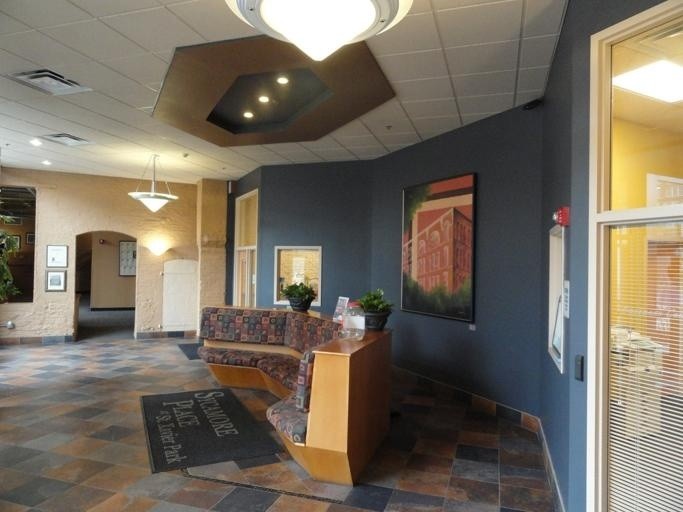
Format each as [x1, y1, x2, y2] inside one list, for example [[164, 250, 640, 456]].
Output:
[[342, 302, 365, 342]]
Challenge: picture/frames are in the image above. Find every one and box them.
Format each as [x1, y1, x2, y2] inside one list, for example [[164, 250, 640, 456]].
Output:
[[44, 245, 69, 293], [273, 245, 323, 306], [4, 216, 35, 249], [399, 172, 476, 323], [118, 240, 136, 278]]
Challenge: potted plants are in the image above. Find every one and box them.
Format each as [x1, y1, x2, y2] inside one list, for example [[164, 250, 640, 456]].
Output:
[[354, 288, 394, 331], [280, 282, 318, 312]]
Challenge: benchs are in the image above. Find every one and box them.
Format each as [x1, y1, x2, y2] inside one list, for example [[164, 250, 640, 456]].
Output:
[[196, 305, 392, 487]]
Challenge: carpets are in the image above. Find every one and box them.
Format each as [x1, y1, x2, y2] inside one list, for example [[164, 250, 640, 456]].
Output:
[[140, 387, 286, 474]]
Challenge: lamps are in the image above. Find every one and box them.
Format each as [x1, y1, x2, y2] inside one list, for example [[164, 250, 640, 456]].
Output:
[[126, 155, 180, 214]]
